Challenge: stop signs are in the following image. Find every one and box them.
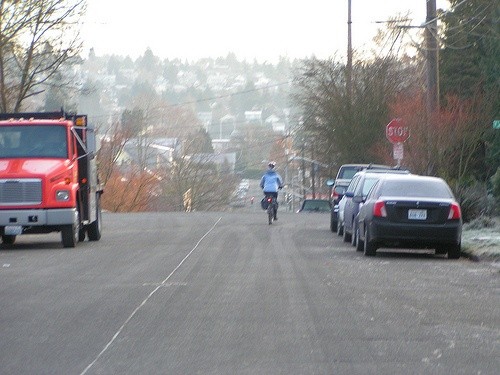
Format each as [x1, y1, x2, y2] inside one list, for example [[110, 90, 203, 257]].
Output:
[[386, 118, 410, 145]]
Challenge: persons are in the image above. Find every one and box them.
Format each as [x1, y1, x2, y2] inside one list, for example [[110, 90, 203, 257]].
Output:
[[259, 161, 284, 221]]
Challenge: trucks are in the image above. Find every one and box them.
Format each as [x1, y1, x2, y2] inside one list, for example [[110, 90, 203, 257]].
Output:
[[0, 108, 104, 249]]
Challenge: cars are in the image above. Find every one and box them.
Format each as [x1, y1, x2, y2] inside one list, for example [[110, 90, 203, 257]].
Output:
[[325, 163, 394, 232], [337, 170, 413, 246], [351, 175, 463, 260]]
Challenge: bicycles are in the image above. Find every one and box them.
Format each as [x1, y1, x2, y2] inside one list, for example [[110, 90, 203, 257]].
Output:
[[266, 198, 275, 224]]
